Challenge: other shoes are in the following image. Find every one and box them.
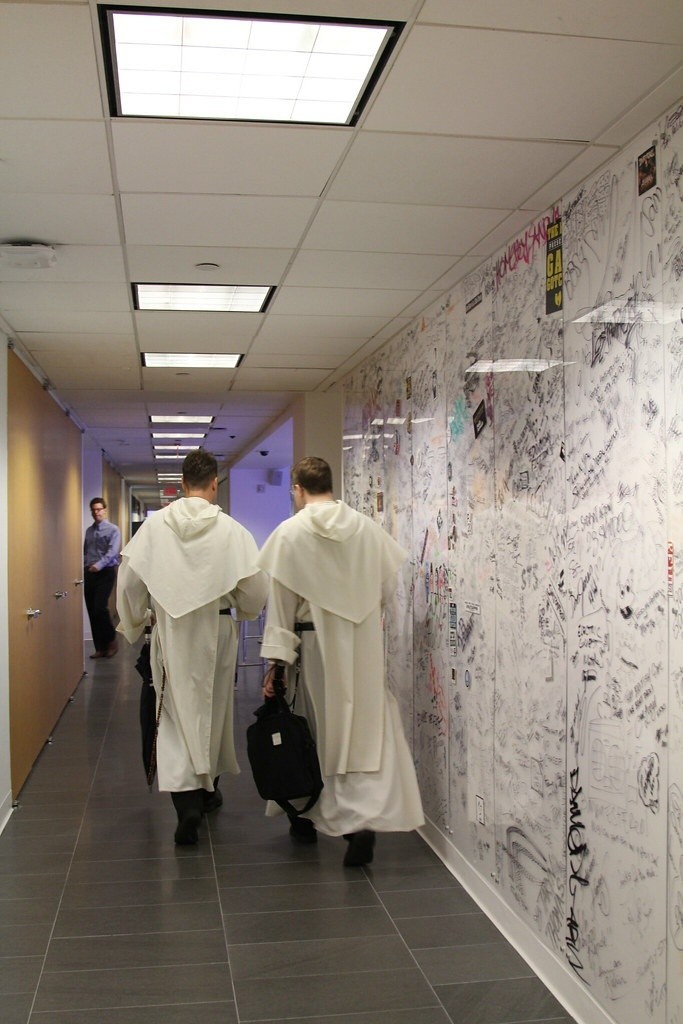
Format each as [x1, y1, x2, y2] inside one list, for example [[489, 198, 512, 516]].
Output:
[[289, 824, 316, 844], [345, 837, 373, 869], [205, 789, 222, 813], [175, 828, 197, 845]]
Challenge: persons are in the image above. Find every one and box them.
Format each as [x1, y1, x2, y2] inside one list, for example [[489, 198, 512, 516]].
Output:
[[84, 498, 121, 660], [115, 446, 270, 845], [255, 457, 425, 870]]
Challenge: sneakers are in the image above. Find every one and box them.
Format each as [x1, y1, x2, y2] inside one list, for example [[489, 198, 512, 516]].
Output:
[[90, 652, 104, 657], [106, 641, 118, 657]]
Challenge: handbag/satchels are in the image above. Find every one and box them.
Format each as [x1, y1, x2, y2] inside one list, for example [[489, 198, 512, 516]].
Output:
[[247, 695, 324, 816]]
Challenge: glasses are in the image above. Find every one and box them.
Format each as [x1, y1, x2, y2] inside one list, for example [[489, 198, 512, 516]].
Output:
[[90, 508, 105, 511]]
